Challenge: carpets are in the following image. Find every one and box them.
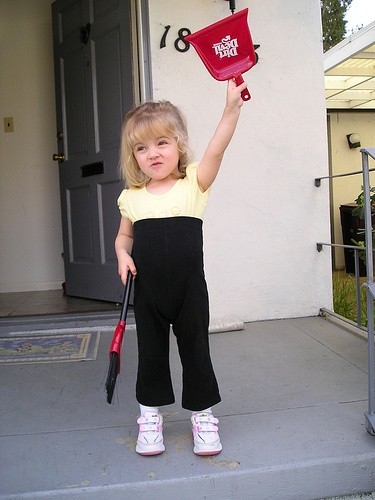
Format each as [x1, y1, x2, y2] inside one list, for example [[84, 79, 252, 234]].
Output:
[[0, 331, 101, 366]]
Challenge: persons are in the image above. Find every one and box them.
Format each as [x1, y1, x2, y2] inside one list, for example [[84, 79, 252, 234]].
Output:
[[115, 77, 249, 456]]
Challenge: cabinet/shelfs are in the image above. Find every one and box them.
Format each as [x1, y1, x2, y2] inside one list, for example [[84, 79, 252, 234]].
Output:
[[339, 203, 375, 277]]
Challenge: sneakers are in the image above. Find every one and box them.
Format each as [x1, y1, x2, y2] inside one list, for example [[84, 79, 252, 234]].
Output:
[[136, 412, 165, 455], [190, 411, 222, 456]]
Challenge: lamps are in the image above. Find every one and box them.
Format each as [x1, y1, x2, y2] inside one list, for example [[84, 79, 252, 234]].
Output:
[[346, 133, 361, 148]]
